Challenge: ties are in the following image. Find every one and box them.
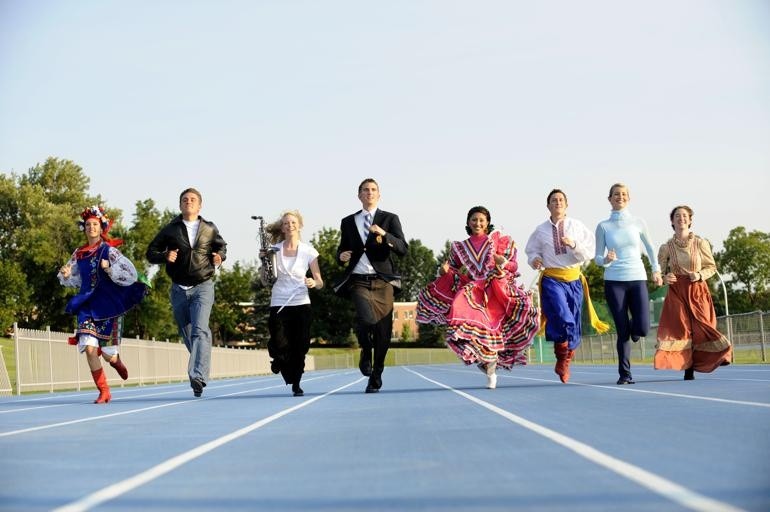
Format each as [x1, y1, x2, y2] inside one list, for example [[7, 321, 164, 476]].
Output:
[[363, 212, 371, 245]]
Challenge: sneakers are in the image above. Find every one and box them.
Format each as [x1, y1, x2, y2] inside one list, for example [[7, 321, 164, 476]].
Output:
[[292, 384, 304, 396], [617, 376, 631, 385], [271, 359, 280, 374], [191, 379, 203, 397], [684, 367, 695, 380]]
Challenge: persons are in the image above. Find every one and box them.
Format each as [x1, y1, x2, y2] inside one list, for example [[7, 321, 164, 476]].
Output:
[[57, 207, 138, 404], [259, 212, 323, 396], [439, 206, 517, 389], [146, 189, 227, 397], [659, 207, 730, 381], [526, 190, 597, 383], [595, 184, 663, 385], [337, 179, 408, 392]]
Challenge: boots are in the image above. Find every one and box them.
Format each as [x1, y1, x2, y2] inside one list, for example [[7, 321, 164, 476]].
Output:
[[554, 340, 576, 384], [110, 353, 128, 380], [91, 367, 111, 403], [486, 362, 497, 389]]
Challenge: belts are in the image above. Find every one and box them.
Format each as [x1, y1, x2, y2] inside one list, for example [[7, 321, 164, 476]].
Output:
[[350, 273, 380, 281]]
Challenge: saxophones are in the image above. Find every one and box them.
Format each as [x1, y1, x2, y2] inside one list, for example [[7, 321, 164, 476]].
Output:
[[251, 216, 281, 290]]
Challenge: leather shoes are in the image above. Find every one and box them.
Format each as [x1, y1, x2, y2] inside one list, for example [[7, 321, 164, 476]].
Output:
[[365, 372, 383, 393], [359, 350, 372, 376]]
